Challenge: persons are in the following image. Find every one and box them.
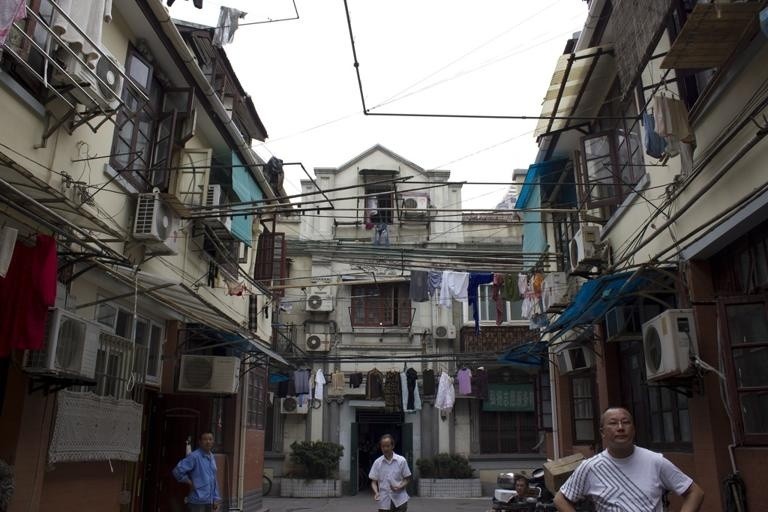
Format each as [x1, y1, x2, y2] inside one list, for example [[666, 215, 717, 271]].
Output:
[[368, 434, 412, 512], [172, 431, 222, 512], [552, 406, 705, 512], [507, 478, 539, 509]]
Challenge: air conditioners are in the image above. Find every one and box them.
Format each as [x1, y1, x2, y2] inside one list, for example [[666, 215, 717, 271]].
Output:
[[177, 353, 241, 394], [206, 184, 233, 231], [304, 293, 333, 352], [279, 395, 310, 414], [541, 226, 602, 313], [642, 309, 702, 381], [133, 192, 181, 257], [65, 35, 126, 115], [432, 324, 457, 340], [24, 306, 102, 380], [401, 195, 428, 215]]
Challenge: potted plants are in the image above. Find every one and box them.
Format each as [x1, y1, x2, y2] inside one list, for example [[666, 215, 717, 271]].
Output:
[[280, 439, 345, 499], [415, 451, 481, 499]]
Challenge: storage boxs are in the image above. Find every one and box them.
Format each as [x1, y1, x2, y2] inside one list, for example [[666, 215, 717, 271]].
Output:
[[542, 451, 589, 496]]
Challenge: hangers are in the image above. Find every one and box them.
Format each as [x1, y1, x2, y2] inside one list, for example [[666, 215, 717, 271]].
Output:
[[641, 79, 688, 114]]
